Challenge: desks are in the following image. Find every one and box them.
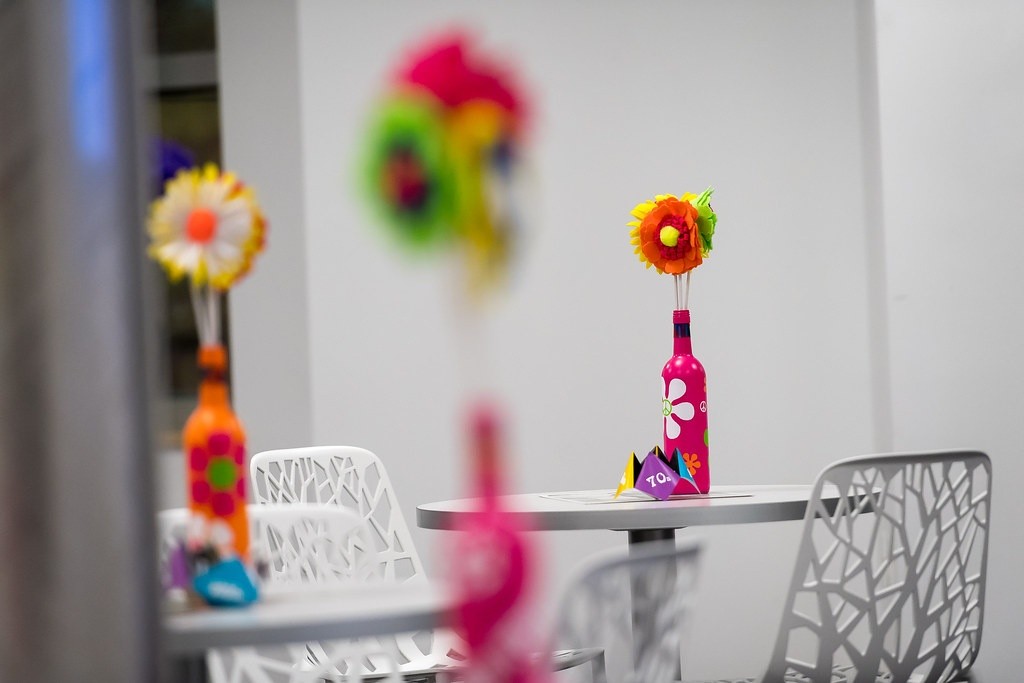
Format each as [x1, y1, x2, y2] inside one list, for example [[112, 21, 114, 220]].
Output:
[[416, 484, 882, 682]]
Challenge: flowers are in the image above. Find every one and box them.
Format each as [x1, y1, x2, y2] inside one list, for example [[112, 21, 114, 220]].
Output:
[[148, 161, 264, 290], [627, 185, 717, 274]]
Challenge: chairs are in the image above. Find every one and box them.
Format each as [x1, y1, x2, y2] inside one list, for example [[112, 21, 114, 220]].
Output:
[[250, 446, 606, 683], [164, 504, 402, 683], [762, 450, 993, 682]]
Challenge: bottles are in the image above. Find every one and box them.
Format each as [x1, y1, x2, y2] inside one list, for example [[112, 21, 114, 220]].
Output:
[[182, 344, 246, 563], [662, 308, 711, 495]]
